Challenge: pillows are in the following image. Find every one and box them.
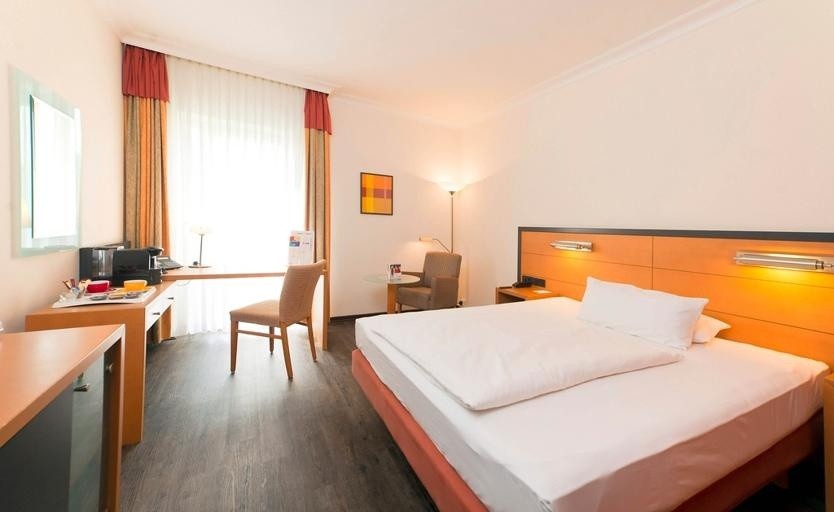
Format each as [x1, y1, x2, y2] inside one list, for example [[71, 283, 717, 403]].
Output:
[[576, 277, 710, 350], [693, 313, 732, 344]]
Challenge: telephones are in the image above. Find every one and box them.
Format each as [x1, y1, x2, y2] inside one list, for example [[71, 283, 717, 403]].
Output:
[[513, 281, 532, 288]]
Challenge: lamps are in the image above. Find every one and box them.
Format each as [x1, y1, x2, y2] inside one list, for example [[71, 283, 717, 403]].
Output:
[[732, 251, 834, 274], [550, 241, 592, 252], [188, 226, 213, 269], [418, 182, 467, 251]]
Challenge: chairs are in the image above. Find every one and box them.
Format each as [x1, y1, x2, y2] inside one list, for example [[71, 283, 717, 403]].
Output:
[[397, 252, 463, 313], [231, 258, 328, 381]]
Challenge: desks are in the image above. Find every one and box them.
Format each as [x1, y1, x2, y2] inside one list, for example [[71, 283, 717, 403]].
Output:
[[24, 266, 329, 448], [363, 274, 420, 313], [0, 323, 126, 512]]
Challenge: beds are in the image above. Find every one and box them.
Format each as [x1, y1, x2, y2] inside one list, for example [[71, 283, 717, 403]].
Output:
[[351, 226, 834, 512]]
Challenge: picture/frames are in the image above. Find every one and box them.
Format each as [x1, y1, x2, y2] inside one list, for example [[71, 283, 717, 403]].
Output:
[[360, 172, 393, 215]]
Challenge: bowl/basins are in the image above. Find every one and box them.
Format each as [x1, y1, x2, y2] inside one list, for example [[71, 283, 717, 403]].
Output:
[[86, 280, 109, 292]]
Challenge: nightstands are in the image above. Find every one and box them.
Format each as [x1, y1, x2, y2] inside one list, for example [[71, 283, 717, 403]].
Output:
[[823, 372, 834, 512], [495, 285, 558, 304]]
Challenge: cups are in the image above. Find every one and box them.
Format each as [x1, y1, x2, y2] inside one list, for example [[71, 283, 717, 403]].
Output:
[[123, 280, 147, 291]]
